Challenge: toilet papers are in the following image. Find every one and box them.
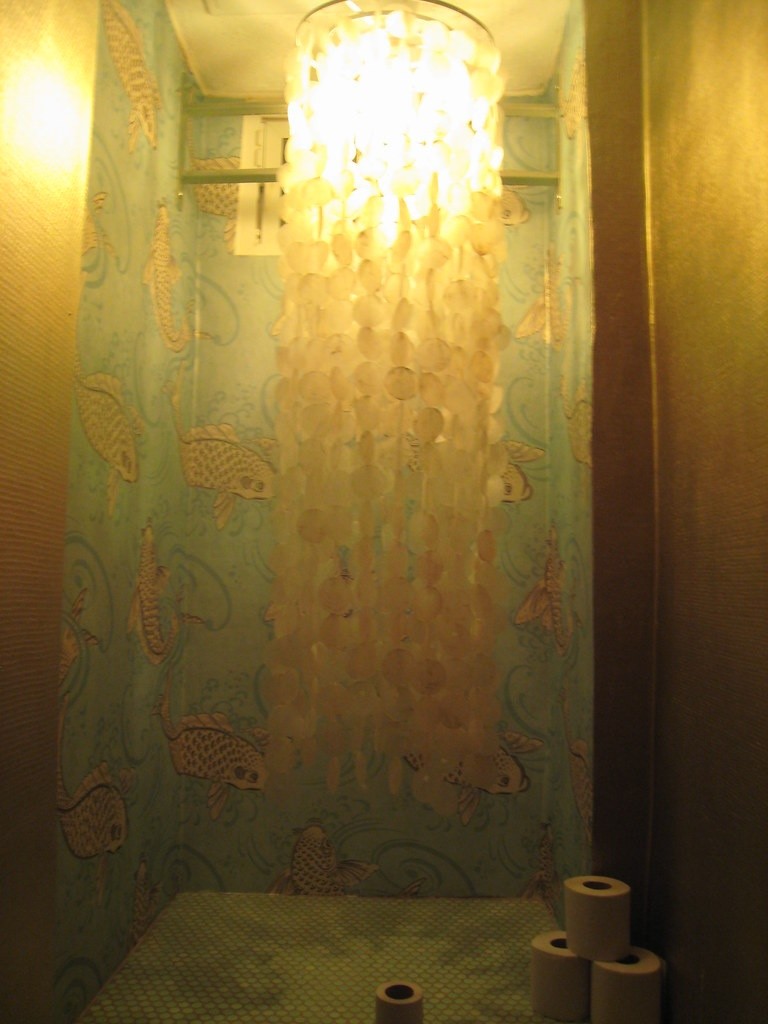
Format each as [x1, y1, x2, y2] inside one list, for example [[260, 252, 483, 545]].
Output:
[[531, 874, 663, 1024], [375, 979, 425, 1024]]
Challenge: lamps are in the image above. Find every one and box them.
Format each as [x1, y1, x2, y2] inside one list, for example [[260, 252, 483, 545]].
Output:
[[284, 0, 506, 246]]
